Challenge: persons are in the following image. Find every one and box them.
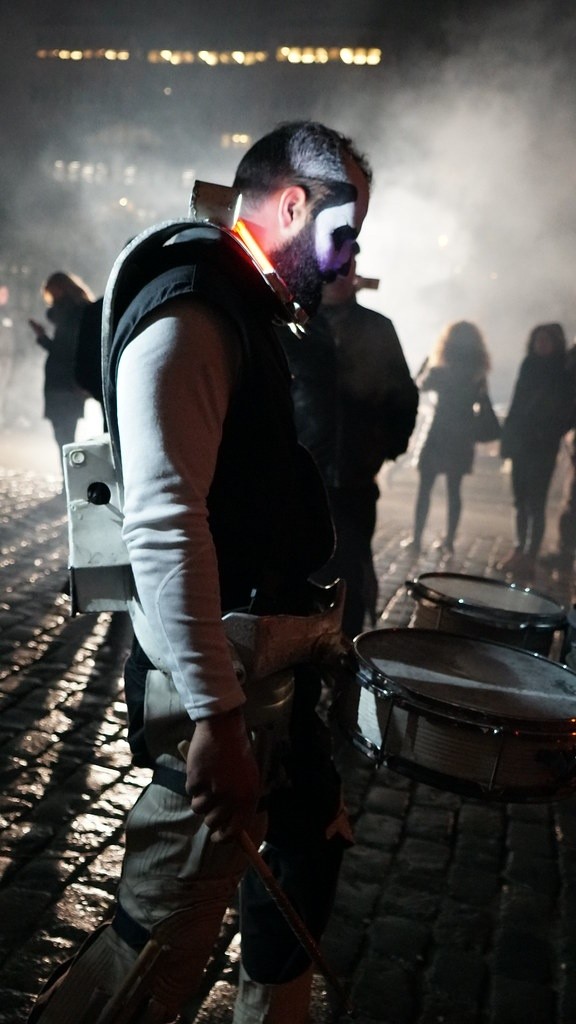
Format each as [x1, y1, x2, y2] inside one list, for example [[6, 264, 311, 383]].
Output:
[[400, 321, 501, 561], [494, 323, 576, 579], [291, 237, 420, 778], [20, 121, 374, 1024], [27, 270, 95, 500]]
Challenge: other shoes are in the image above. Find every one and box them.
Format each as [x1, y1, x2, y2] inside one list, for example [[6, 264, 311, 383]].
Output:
[[540, 550, 575, 570], [434, 540, 454, 555], [499, 543, 534, 579], [400, 538, 419, 550]]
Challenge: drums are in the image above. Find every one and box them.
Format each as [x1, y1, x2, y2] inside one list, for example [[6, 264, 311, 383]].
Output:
[[352, 629, 576, 806], [405, 571, 560, 659]]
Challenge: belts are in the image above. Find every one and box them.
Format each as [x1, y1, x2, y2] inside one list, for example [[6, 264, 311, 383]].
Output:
[[219, 584, 339, 617]]
